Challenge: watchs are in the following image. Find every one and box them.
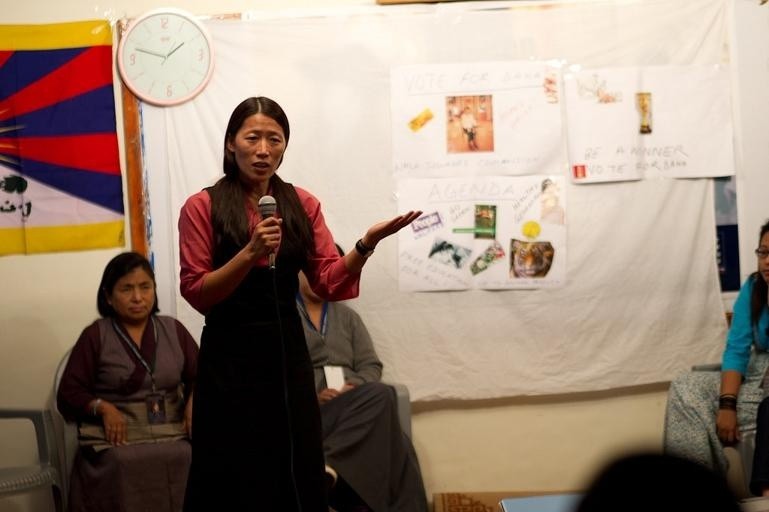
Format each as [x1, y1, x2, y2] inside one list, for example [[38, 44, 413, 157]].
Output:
[[356, 239, 374, 258]]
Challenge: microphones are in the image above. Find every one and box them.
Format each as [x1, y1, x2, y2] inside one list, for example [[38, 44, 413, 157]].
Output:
[[259, 193, 278, 274]]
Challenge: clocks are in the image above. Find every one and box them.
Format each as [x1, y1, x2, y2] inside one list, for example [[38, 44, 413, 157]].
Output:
[[116, 7, 215, 107]]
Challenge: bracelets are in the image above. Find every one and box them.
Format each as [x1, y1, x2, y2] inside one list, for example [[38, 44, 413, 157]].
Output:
[[719, 393, 737, 411], [93, 398, 101, 414]]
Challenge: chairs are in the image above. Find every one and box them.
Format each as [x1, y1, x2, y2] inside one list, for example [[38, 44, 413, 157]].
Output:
[[0, 346, 78, 512]]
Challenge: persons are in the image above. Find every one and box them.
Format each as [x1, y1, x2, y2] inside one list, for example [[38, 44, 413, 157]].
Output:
[[175, 97, 424, 509], [749, 397, 769, 497], [715, 218, 769, 443], [57, 251, 200, 511], [296, 244, 427, 511], [576, 450, 745, 511], [449, 96, 487, 151]]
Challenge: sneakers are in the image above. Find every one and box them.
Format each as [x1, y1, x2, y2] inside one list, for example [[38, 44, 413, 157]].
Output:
[[325, 463, 339, 495]]
